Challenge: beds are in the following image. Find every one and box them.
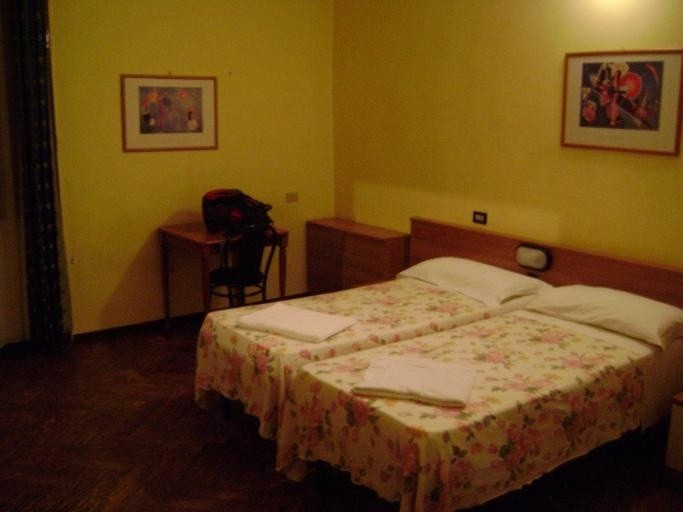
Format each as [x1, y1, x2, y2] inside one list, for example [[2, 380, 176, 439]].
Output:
[[277, 281, 683, 508], [201, 278, 566, 479]]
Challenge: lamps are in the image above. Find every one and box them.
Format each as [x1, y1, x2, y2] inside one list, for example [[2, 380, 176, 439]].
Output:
[[514, 245, 552, 271]]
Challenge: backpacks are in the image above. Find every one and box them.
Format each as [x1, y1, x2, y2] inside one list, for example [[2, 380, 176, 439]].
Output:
[[202, 188, 273, 236]]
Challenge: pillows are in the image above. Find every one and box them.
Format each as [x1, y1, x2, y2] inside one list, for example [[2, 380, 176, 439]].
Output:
[[524, 278, 681, 353], [397, 255, 551, 310]]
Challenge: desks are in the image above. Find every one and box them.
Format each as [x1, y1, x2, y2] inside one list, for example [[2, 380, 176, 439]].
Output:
[[157, 224, 289, 329]]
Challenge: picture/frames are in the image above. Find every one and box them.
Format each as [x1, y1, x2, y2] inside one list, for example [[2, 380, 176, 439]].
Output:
[[559, 49, 682, 157], [119, 74, 219, 152]]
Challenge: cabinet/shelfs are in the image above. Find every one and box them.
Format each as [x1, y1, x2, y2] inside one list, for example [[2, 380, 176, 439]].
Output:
[[305, 217, 410, 295]]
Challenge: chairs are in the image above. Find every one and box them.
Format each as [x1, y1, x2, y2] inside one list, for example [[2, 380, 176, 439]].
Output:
[[209, 222, 278, 309]]
